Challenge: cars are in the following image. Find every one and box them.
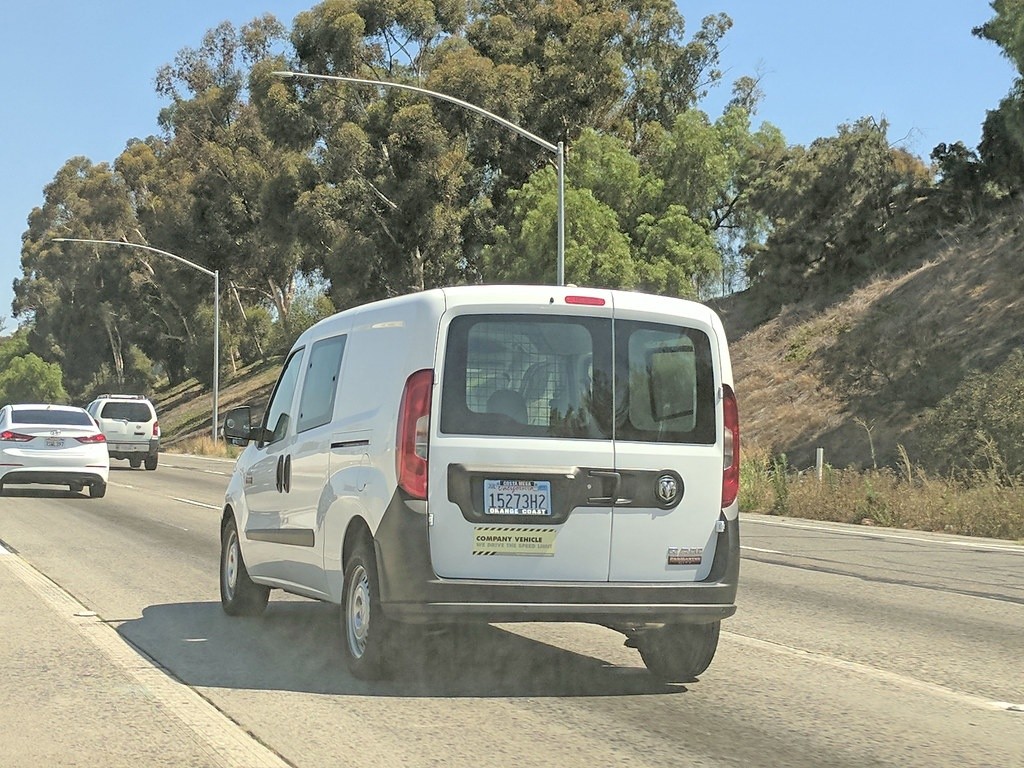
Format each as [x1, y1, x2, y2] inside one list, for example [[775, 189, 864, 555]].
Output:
[[1, 405, 110, 498]]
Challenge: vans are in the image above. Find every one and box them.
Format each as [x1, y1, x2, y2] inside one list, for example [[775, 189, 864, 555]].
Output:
[[218, 284, 746, 686]]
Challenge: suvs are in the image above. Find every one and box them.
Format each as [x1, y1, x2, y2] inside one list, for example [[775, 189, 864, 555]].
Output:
[[82, 393, 161, 473]]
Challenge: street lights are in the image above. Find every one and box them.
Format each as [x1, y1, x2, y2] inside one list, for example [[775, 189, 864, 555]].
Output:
[[273, 69, 566, 283], [50, 236, 217, 444]]
